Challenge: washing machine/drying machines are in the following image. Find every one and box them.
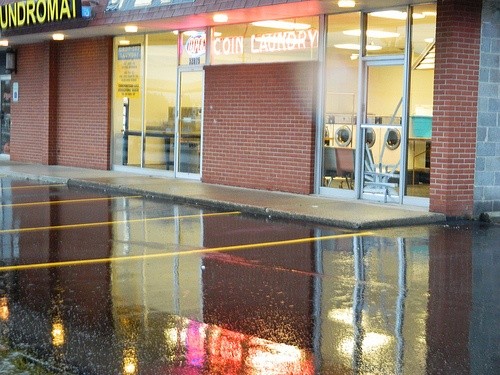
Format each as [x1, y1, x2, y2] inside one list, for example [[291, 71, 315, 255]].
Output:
[[379, 123, 428, 172], [325, 122, 334, 147], [351, 123, 379, 172], [332, 122, 353, 153]]
